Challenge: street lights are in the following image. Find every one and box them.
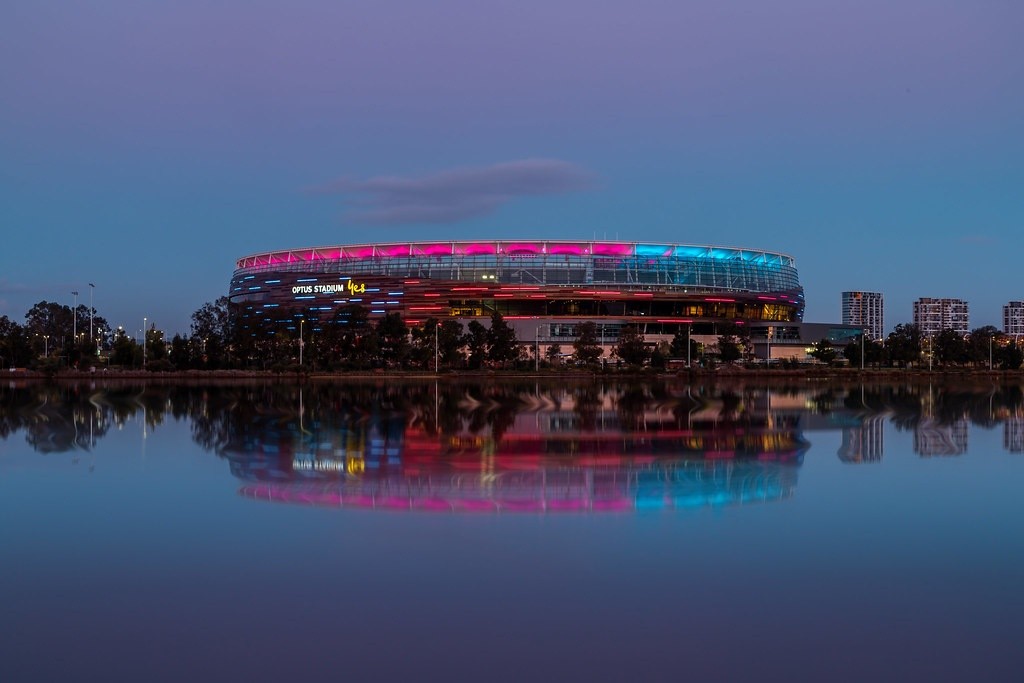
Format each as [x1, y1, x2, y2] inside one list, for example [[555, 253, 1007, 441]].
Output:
[[300, 320, 305, 365], [44, 334, 49, 359], [862, 332, 866, 369], [990, 334, 995, 370], [535, 324, 544, 371], [767, 328, 786, 368], [71, 290, 78, 343], [89, 283, 94, 342], [687, 324, 693, 369], [930, 333, 934, 371], [602, 328, 607, 370], [436, 322, 442, 373], [143, 318, 147, 364]]
